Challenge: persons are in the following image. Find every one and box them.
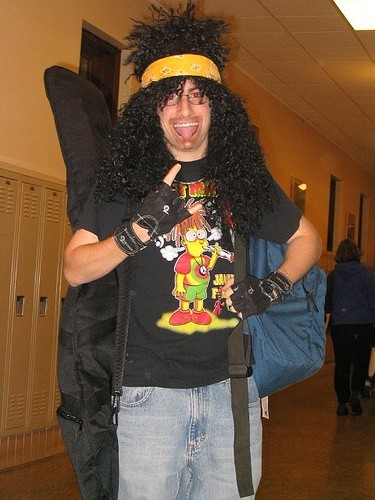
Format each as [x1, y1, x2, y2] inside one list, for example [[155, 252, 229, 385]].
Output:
[[323, 238, 374, 416], [62, 7, 324, 500]]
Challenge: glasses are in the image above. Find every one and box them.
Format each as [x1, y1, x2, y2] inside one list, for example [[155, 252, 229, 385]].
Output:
[[158, 90, 213, 106]]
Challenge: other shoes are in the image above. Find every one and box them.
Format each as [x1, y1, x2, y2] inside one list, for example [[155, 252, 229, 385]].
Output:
[[350, 398, 362, 416], [337, 404, 350, 417]]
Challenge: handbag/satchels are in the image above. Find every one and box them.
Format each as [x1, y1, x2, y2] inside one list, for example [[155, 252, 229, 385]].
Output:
[[247, 235, 328, 399]]
